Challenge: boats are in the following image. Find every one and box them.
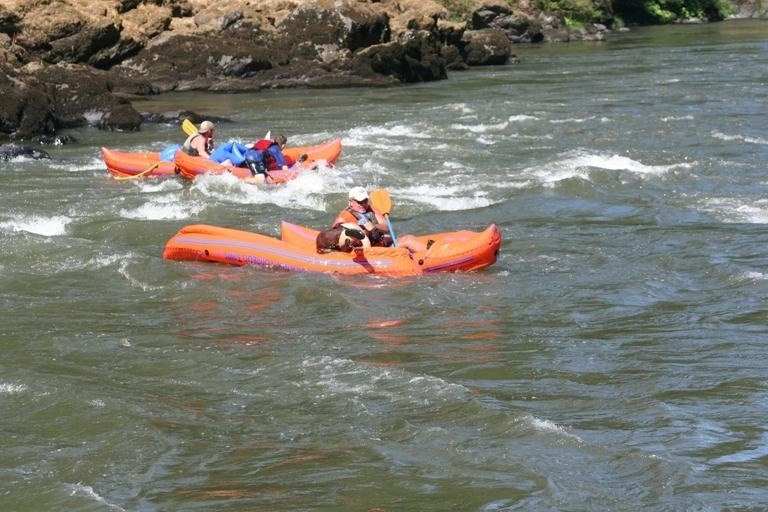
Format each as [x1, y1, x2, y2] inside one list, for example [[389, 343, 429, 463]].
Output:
[[100, 144, 251, 183], [172, 136, 341, 191], [160, 222, 422, 281], [279, 221, 502, 275]]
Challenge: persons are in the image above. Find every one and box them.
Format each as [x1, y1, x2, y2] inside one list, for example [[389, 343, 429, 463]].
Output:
[[252, 133, 292, 171], [181, 118, 220, 158], [335, 187, 425, 253]]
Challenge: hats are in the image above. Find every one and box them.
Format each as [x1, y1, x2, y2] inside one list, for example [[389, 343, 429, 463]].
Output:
[[199, 121, 214, 133], [349, 186, 368, 202]]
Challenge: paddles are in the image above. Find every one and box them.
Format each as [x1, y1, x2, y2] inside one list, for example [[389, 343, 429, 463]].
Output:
[[371, 191, 398, 248], [182, 119, 198, 135]]
